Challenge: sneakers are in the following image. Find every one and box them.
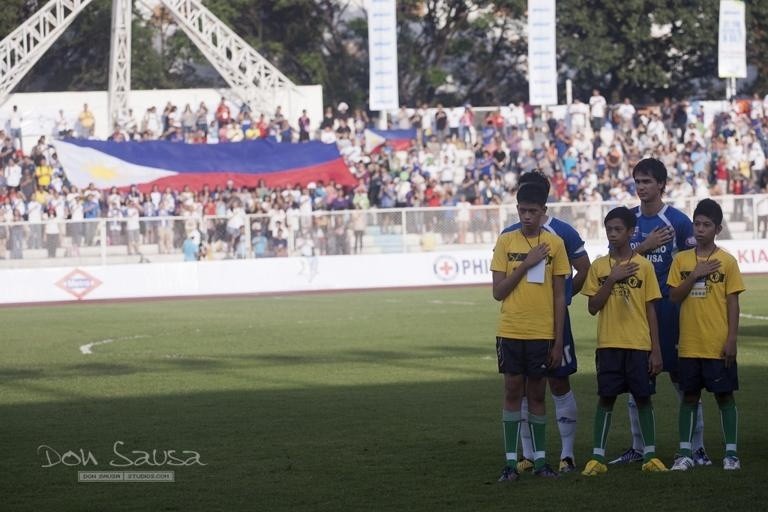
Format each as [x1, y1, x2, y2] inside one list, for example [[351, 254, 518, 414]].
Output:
[[723, 455, 740, 471], [497, 446, 713, 483]]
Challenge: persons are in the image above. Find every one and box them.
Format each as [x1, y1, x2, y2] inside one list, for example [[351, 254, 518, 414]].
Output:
[[579, 204, 669, 476], [664, 198, 747, 471], [488, 181, 573, 481], [608, 158, 714, 469], [0, 85, 768, 262], [499, 172, 592, 476]]
